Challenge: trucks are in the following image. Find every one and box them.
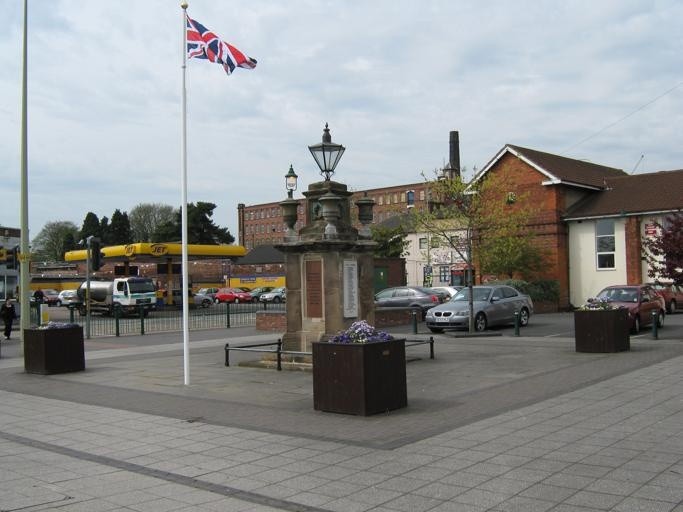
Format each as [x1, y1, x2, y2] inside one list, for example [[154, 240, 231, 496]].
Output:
[[77, 276, 157, 317]]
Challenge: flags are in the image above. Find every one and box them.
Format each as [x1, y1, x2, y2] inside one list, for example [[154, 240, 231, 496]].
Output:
[[184, 11, 257, 76]]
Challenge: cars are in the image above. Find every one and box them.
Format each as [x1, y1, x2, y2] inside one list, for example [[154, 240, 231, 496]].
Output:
[[373, 286, 444, 319], [644, 282, 682, 314], [55, 289, 82, 307], [425, 284, 534, 332], [163, 287, 285, 308], [40, 289, 58, 305], [587, 284, 666, 334], [29, 290, 40, 305], [429, 286, 465, 301]]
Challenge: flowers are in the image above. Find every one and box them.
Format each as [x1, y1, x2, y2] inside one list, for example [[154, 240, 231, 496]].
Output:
[[327, 319, 392, 344], [578, 297, 624, 310], [31, 321, 72, 330]]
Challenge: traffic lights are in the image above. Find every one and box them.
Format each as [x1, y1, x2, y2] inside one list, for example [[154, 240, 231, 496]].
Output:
[[91, 238, 105, 271], [6, 248, 16, 270]]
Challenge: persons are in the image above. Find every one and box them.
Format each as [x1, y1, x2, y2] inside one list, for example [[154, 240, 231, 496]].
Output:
[[34, 287, 46, 314], [0, 297, 18, 340]]
